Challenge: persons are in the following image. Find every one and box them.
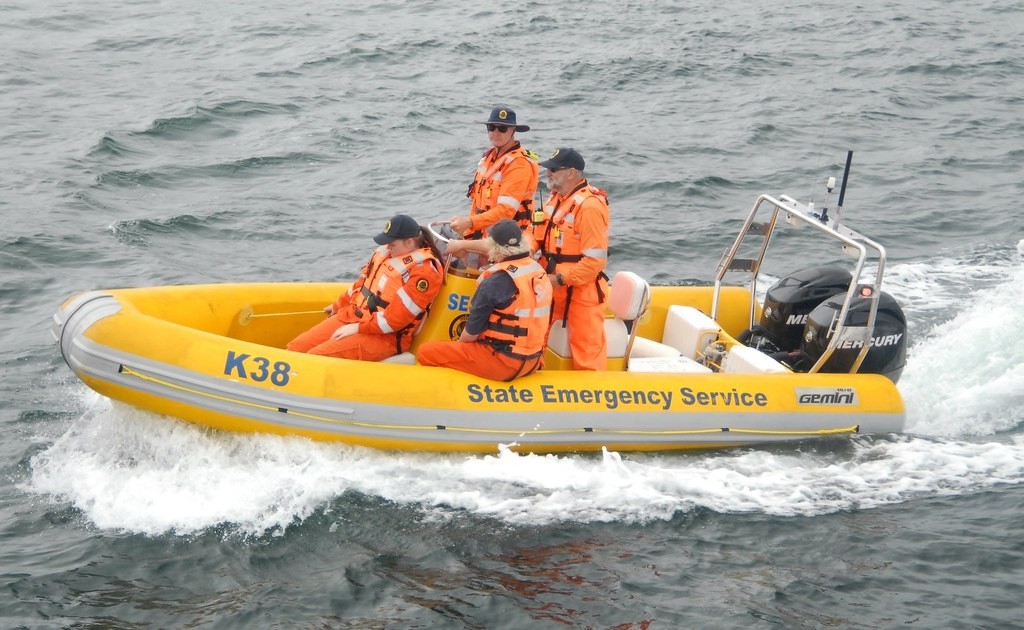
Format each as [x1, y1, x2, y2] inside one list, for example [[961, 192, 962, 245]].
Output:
[[525, 148, 608, 371], [287, 215, 444, 363], [416, 219, 553, 382], [449, 108, 538, 241]]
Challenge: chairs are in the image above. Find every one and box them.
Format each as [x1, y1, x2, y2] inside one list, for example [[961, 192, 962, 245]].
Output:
[[661, 304, 721, 362], [724, 345, 789, 376], [378, 296, 435, 365], [547, 271, 651, 358]]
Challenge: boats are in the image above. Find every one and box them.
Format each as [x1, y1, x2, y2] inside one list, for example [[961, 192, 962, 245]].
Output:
[[50, 148, 908, 456]]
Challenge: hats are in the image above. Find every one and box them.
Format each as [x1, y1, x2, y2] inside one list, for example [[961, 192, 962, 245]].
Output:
[[484, 106, 530, 132], [485, 220, 523, 247], [372, 215, 421, 245], [537, 147, 586, 173]]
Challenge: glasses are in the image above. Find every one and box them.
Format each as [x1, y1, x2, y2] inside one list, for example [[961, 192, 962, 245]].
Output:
[[550, 167, 570, 172], [487, 124, 514, 133]]
